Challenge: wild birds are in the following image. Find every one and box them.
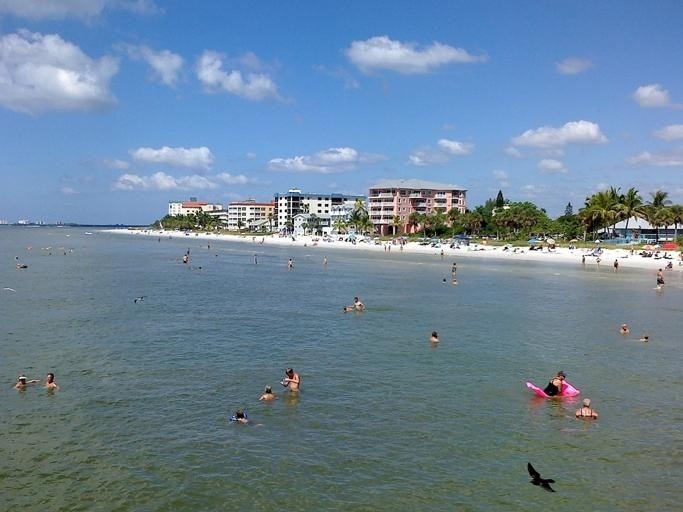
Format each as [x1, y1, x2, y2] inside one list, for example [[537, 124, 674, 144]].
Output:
[[133, 294, 145, 304], [527, 462, 556, 493]]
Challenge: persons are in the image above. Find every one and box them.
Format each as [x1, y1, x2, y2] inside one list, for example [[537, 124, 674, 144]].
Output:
[[580, 254, 586, 266], [612, 258, 618, 273], [441, 277, 447, 284], [342, 307, 352, 313], [348, 296, 367, 312], [428, 329, 440, 343], [182, 248, 190, 264], [617, 322, 631, 335], [283, 367, 300, 392], [286, 258, 294, 265], [11, 375, 39, 391], [43, 373, 60, 390], [288, 263, 295, 271], [253, 253, 257, 265], [322, 255, 327, 264], [258, 384, 278, 401], [575, 398, 597, 422], [664, 261, 672, 271], [235, 410, 265, 429], [542, 370, 566, 397], [654, 267, 664, 289], [595, 257, 601, 267], [449, 261, 456, 277], [639, 335, 648, 344], [450, 278, 459, 286]]
